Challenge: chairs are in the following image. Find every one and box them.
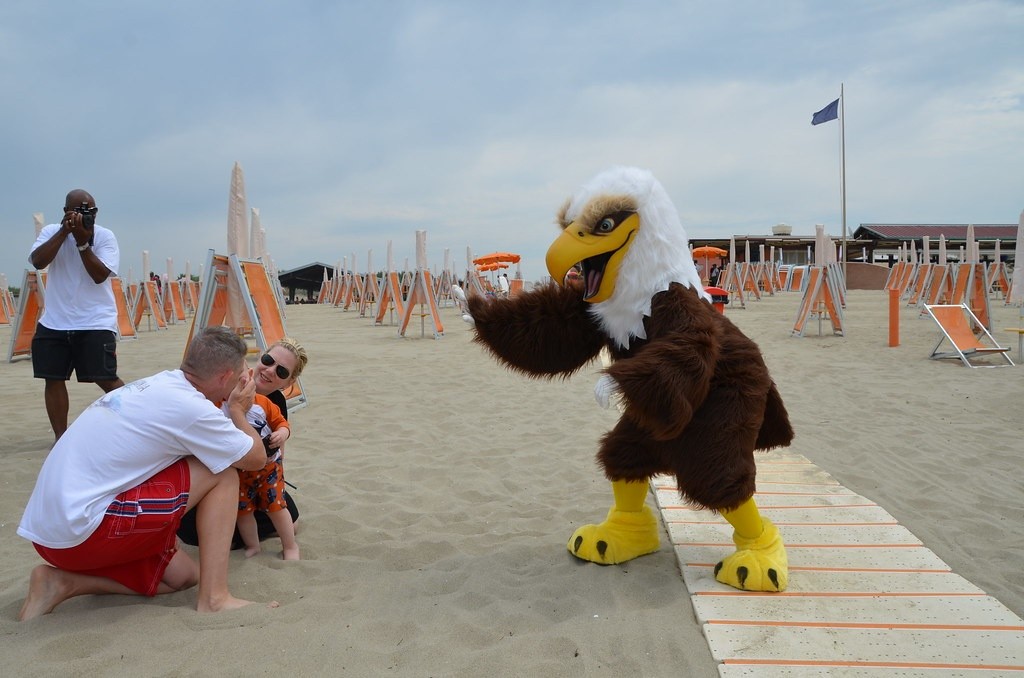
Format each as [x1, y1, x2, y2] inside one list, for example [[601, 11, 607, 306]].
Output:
[[923, 303, 1015, 369]]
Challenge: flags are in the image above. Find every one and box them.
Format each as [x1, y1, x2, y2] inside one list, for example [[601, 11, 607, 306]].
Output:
[[812, 98, 839, 125]]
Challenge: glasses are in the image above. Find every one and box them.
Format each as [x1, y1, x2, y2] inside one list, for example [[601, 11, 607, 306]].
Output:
[[261, 354, 290, 379]]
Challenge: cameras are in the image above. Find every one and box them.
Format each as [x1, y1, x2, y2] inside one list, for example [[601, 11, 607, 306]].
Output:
[[73, 202, 98, 228]]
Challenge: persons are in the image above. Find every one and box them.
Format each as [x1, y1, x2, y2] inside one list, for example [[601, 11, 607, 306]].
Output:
[[710, 263, 721, 287], [214, 359, 300, 562], [314, 296, 318, 304], [150, 272, 161, 303], [497, 275, 502, 292], [26, 188, 125, 440], [174, 336, 308, 550], [694, 260, 701, 277], [16, 325, 279, 623], [501, 274, 511, 297]]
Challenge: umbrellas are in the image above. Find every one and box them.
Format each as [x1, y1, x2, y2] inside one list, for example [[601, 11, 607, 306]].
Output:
[[473, 251, 521, 275], [693, 244, 728, 279], [477, 262, 509, 283]]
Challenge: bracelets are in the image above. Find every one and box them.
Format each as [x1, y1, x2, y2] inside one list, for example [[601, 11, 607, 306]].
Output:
[[77, 241, 89, 251]]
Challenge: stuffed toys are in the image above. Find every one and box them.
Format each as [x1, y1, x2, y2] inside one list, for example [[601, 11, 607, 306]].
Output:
[[450, 164, 795, 592]]
